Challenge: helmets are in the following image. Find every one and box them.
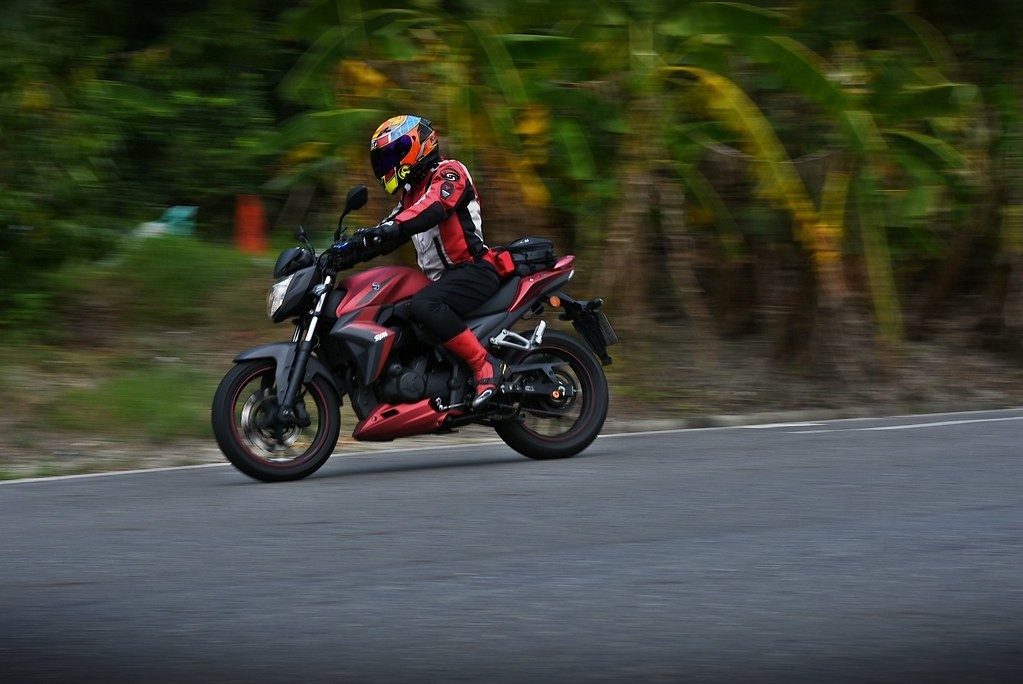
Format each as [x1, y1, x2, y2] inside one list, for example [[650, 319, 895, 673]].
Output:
[[370, 115, 441, 195]]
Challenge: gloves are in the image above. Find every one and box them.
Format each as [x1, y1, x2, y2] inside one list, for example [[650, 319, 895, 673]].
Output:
[[354, 221, 401, 257], [319, 245, 356, 278]]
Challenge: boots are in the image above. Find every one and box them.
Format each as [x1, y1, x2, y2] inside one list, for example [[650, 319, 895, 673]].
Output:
[[444, 326, 505, 406]]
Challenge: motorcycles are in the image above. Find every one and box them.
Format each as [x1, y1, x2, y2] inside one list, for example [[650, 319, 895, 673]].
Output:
[[210, 187, 616, 484]]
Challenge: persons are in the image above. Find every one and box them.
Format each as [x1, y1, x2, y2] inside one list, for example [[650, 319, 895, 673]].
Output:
[[334, 114, 514, 410]]
